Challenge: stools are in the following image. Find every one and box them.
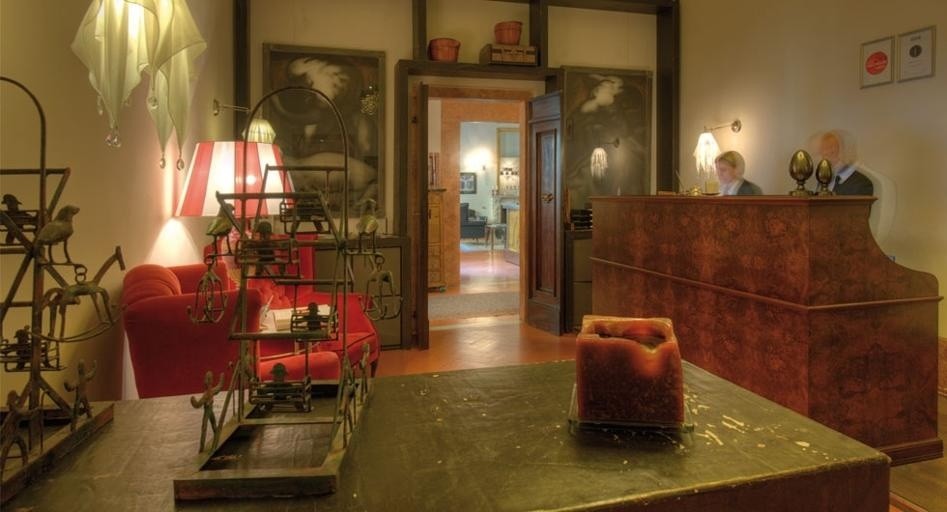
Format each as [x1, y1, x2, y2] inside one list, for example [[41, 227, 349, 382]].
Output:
[[484, 223, 507, 250]]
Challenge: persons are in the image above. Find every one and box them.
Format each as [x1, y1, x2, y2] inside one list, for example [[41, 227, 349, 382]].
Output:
[[713, 151, 762, 196], [805, 131, 872, 219]]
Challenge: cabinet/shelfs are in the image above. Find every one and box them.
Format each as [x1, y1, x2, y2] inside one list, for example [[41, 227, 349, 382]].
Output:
[[428, 188, 447, 291], [564, 229, 594, 330]]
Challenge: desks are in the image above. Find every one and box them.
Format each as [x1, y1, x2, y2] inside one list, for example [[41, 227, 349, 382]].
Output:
[[1, 358, 893, 508]]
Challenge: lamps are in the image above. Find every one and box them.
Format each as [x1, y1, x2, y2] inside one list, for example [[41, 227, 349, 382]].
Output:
[[68, 0, 206, 173], [213, 100, 277, 143], [694, 119, 741, 174], [590, 137, 621, 181], [173, 142, 300, 256]]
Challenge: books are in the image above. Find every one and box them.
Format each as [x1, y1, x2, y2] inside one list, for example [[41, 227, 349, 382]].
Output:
[[272, 304, 338, 332]]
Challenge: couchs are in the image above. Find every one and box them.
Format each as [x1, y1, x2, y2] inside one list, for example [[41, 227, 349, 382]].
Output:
[[201, 234, 379, 380], [459, 202, 486, 243], [121, 260, 342, 397]]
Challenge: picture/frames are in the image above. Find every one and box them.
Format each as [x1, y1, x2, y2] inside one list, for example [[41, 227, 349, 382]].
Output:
[[560, 64, 652, 211], [856, 36, 896, 89], [459, 172, 480, 194], [258, 38, 390, 222], [897, 25, 937, 84]]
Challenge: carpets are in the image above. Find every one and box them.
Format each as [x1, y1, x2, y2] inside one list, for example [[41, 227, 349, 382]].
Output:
[[888, 489, 929, 511]]
